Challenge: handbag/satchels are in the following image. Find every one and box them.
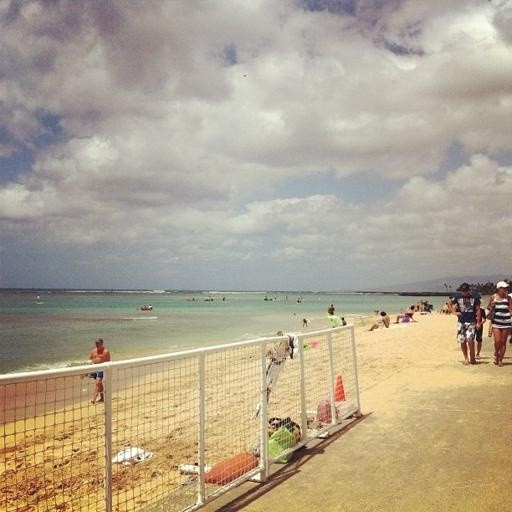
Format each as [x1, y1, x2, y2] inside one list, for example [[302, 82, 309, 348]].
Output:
[[267, 416, 300, 463]]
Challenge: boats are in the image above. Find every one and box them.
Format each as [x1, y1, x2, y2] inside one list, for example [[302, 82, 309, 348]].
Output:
[[137, 306, 153, 312]]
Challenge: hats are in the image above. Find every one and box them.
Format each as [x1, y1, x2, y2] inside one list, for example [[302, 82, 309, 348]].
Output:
[[496, 281, 509, 289], [94, 337, 102, 343], [456, 282, 469, 291]]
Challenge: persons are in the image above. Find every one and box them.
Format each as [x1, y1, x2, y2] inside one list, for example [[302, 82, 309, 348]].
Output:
[[368, 310, 390, 332], [302, 318, 307, 327], [394, 304, 415, 323], [413, 295, 460, 314], [341, 317, 347, 326], [447, 281, 512, 366], [327, 304, 335, 315], [87, 337, 111, 404]]
